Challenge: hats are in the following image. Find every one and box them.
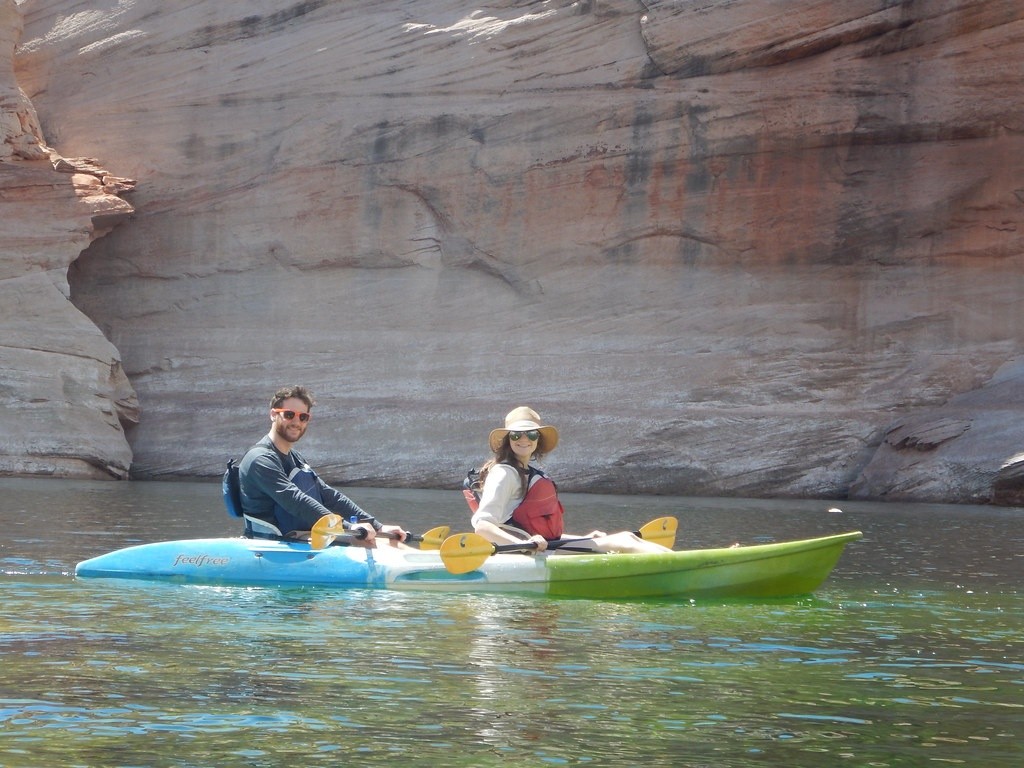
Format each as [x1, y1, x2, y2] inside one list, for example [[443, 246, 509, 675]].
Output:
[[489, 406, 558, 456]]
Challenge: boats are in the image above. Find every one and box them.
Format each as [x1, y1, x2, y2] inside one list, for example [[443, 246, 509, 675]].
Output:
[[68, 528, 865, 596]]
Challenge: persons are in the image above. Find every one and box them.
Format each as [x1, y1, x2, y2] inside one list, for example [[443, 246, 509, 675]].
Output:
[[463, 406, 676, 557], [222, 384, 420, 551]]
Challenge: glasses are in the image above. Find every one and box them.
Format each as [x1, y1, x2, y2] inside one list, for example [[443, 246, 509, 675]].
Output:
[[507, 431, 540, 441], [273, 408, 311, 423]]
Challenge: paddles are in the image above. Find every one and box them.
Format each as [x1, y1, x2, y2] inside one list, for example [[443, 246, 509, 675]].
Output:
[[310, 513, 450, 550], [441, 516, 682, 574]]
